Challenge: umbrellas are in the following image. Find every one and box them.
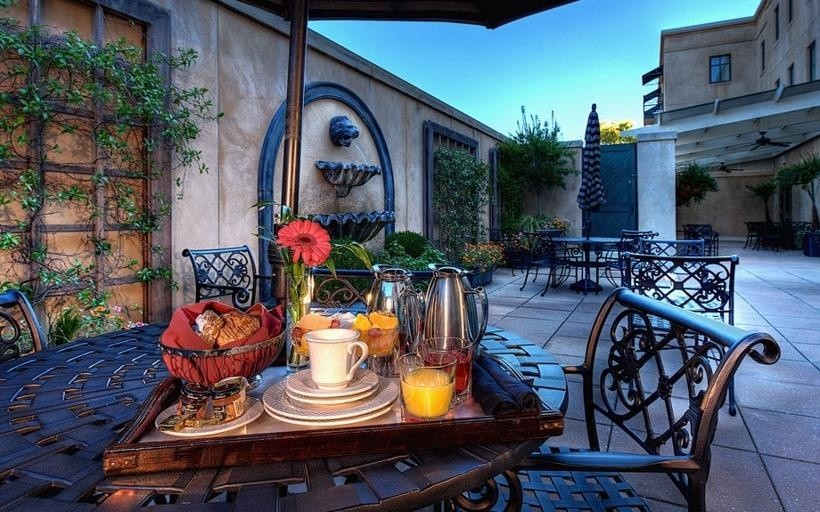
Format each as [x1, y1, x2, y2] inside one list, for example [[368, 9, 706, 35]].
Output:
[[240, 1, 577, 311], [576, 104, 608, 280]]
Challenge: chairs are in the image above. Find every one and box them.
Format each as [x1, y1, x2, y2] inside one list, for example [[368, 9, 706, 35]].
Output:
[[310, 266, 433, 312], [0, 289, 47, 366], [181, 246, 276, 309]]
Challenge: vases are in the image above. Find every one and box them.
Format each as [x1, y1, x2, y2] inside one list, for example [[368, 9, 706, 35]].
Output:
[[283, 273, 315, 373]]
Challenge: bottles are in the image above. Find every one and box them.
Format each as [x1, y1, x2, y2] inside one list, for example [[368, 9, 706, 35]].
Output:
[[177, 378, 248, 420]]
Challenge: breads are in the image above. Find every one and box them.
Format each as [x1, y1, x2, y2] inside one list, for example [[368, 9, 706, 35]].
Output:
[[193, 311, 260, 348]]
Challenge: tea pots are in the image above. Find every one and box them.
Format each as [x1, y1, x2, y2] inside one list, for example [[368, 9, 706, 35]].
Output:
[[425, 261, 489, 356], [367, 263, 422, 378]]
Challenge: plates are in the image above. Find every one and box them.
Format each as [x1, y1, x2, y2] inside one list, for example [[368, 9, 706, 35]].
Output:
[[154, 393, 264, 436], [287, 367, 378, 405], [262, 372, 399, 427]]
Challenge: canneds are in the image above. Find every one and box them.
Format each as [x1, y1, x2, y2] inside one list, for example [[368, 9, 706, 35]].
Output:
[[215, 376, 247, 403], [176, 382, 211, 420], [212, 384, 245, 421]]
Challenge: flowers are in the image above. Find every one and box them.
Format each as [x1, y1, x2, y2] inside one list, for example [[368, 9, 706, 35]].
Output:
[[249, 200, 330, 270]]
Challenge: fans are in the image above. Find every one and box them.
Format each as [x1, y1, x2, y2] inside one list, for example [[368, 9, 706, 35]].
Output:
[[743, 130, 791, 152]]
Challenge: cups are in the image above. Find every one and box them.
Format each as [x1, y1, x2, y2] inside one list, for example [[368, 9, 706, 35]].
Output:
[[421, 336, 473, 401], [399, 351, 457, 419], [304, 327, 369, 391]]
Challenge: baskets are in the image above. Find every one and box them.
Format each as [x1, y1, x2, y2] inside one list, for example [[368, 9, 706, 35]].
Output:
[[156, 319, 289, 387]]
